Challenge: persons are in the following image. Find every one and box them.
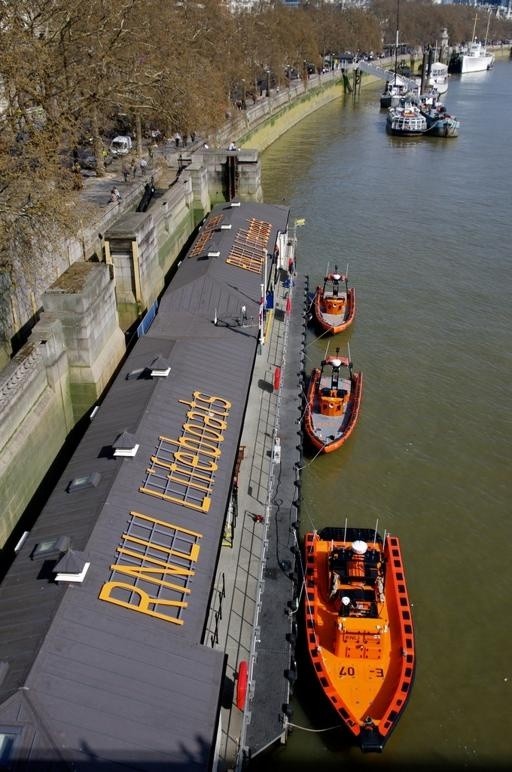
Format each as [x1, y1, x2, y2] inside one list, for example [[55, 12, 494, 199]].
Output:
[[227, 142, 238, 151], [106, 123, 197, 208], [202, 141, 209, 151]]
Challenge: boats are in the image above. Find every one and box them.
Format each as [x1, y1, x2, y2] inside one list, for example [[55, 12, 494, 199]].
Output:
[[386, 90, 427, 136], [303, 340, 364, 453], [315, 261, 356, 334], [418, 82, 460, 138], [301, 517, 416, 754]]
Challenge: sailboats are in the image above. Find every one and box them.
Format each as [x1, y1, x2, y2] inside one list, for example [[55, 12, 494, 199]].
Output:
[[379, 0, 441, 108], [453, 13, 496, 74], [424, 39, 449, 94]]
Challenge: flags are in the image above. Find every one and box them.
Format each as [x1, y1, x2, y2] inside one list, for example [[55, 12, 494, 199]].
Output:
[[295, 219, 305, 227], [258, 296, 265, 330]]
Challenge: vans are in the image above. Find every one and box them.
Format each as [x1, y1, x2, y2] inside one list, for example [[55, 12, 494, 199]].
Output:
[[109, 135, 133, 158]]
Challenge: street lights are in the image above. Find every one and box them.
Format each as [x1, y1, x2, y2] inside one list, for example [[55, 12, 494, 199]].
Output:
[[286, 64, 290, 87], [241, 78, 248, 110], [303, 59, 308, 80], [266, 70, 271, 96]]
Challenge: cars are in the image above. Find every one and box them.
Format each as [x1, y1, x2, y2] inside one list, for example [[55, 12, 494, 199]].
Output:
[[82, 146, 112, 167]]
